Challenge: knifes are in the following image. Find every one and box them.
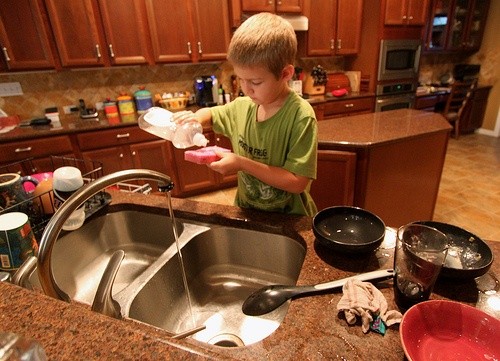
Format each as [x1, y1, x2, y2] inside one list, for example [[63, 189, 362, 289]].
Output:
[[311, 65, 326, 87]]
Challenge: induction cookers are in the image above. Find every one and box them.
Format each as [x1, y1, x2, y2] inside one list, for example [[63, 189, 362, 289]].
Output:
[[241, 12, 309, 33]]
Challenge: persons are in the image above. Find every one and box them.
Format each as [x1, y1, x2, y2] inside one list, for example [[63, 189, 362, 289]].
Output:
[[169, 11, 319, 218]]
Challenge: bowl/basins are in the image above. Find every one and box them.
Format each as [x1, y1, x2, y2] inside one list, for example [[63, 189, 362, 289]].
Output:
[[399, 300, 500, 361], [313, 205, 386, 254], [404, 220, 494, 282]]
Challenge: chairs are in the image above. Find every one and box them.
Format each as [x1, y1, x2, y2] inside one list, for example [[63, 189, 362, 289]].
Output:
[[443, 79, 478, 140]]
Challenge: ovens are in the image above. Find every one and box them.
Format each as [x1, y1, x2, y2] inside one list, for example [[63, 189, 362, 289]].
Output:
[[375, 38, 423, 115]]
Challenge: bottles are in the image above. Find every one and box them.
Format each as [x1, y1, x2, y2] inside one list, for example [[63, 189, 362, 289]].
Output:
[[217, 84, 225, 105], [103, 86, 153, 117], [137, 106, 210, 149]]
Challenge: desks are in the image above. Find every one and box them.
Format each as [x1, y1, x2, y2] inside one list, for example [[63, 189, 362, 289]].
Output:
[[417, 84, 493, 134]]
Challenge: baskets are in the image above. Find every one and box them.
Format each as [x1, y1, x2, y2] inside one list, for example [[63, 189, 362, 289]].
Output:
[[158, 98, 189, 110]]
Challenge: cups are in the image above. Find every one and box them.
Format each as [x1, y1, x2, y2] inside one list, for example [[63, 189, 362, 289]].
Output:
[[51, 166, 86, 231], [393, 223, 448, 312], [0, 173, 39, 215], [0, 212, 38, 271]]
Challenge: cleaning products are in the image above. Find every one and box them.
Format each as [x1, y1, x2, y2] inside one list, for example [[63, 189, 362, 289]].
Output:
[[137, 106, 208, 149]]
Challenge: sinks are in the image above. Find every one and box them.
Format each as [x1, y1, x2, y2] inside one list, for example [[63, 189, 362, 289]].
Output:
[[129, 226, 307, 348], [28, 203, 185, 306]]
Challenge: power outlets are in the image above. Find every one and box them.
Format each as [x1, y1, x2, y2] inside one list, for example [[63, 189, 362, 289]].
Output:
[[63, 105, 77, 114]]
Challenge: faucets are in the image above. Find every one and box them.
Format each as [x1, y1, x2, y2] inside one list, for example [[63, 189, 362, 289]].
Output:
[[36, 168, 175, 302]]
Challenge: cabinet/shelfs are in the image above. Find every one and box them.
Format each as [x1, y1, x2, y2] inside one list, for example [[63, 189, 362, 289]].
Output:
[[170, 120, 238, 197], [363, 0, 427, 26], [309, 130, 450, 229], [241, 0, 304, 14], [141, 0, 241, 65], [41, 0, 151, 70], [324, 96, 373, 119], [294, 0, 363, 59], [0, 134, 85, 177], [0, 0, 59, 73], [420, 0, 491, 53], [73, 126, 181, 198]]
